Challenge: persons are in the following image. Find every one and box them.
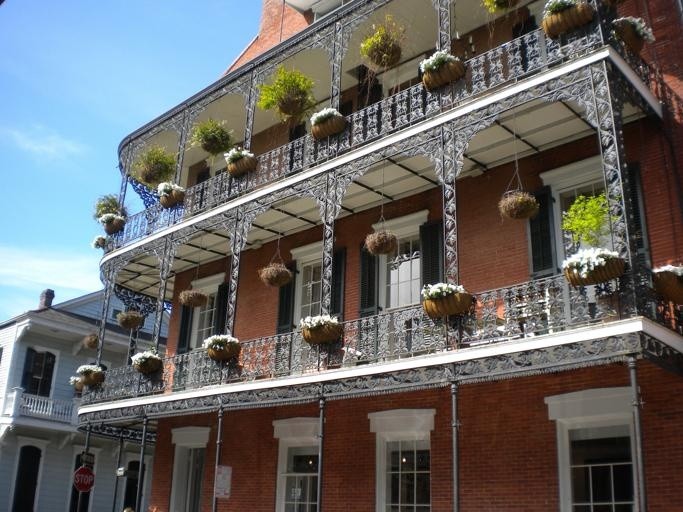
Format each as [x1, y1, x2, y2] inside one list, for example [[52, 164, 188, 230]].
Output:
[[123, 507, 134, 512], [512, 5, 537, 72]]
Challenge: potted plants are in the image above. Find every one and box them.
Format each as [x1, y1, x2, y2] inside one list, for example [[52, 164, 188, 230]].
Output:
[[497, 191, 539, 219], [366, 232, 396, 255], [191, 117, 231, 155], [259, 264, 291, 288], [84, 334, 99, 350], [255, 64, 315, 120], [179, 291, 206, 309], [132, 144, 176, 186], [117, 311, 144, 330], [94, 196, 124, 214], [360, 26, 402, 66]]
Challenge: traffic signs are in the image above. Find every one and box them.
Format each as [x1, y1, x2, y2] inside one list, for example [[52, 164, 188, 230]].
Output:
[[79, 451, 95, 465]]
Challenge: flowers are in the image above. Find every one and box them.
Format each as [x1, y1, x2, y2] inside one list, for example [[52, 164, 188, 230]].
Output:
[[97, 213, 113, 225], [223, 146, 257, 165], [130, 348, 161, 365], [172, 184, 185, 192], [420, 282, 465, 300], [68, 376, 83, 386], [308, 107, 343, 126], [298, 314, 340, 331], [200, 333, 240, 350], [156, 181, 173, 199], [541, 0, 574, 20], [611, 15, 655, 44], [560, 246, 619, 280], [113, 215, 124, 220], [418, 51, 460, 72], [77, 364, 104, 375], [653, 264, 683, 278], [92, 235, 107, 249]]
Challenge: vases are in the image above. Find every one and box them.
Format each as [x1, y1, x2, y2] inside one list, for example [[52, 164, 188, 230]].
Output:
[[226, 156, 258, 177], [132, 357, 162, 377], [301, 323, 342, 345], [80, 371, 105, 386], [113, 220, 124, 229], [103, 220, 114, 234], [653, 270, 682, 306], [171, 190, 184, 204], [422, 292, 474, 320], [310, 116, 347, 141], [614, 19, 645, 54], [207, 343, 240, 363], [563, 257, 627, 289], [421, 60, 466, 90], [159, 194, 175, 209], [543, 4, 592, 38]]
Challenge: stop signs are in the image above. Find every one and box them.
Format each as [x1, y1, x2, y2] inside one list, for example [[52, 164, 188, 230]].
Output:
[[74, 467, 94, 492]]
